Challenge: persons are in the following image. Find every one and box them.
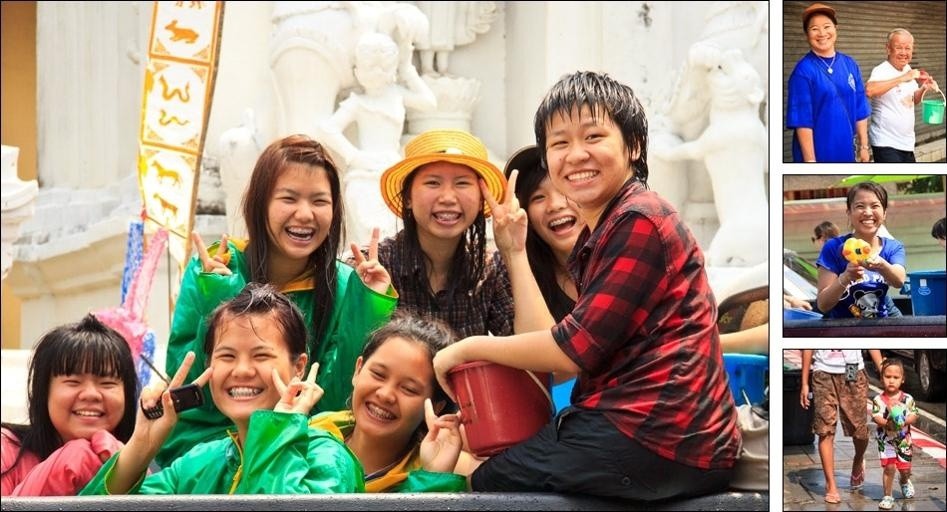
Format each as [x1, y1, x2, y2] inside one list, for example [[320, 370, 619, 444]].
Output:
[[864, 28, 940, 163], [419, 70, 745, 504], [798, 348, 884, 506], [0, 310, 134, 499], [871, 357, 921, 510], [810, 216, 947, 254], [164, 132, 589, 419], [70, 278, 366, 493], [307, 313, 469, 493], [817, 181, 907, 319], [784, 4, 870, 164], [718, 322, 769, 357]]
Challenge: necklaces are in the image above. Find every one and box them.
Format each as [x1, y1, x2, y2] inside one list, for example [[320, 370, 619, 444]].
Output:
[[818, 53, 836, 75]]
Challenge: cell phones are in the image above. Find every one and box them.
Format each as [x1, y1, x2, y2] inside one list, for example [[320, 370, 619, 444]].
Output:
[[141, 384, 205, 420]]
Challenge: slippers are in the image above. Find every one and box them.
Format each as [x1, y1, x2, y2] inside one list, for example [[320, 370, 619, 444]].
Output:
[[900, 479, 914, 499], [851, 460, 865, 490], [825, 492, 840, 503], [879, 496, 895, 509]]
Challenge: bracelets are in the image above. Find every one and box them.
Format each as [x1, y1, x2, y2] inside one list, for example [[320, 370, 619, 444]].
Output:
[[837, 272, 853, 288], [859, 145, 870, 151]]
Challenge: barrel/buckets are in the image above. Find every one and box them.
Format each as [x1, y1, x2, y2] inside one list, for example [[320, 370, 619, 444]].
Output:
[[910, 270, 944, 316], [448, 362, 553, 453], [921, 89, 946, 125]]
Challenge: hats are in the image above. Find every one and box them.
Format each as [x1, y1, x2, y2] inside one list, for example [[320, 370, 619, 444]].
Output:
[[380, 130, 507, 218], [803, 3, 836, 23]]
